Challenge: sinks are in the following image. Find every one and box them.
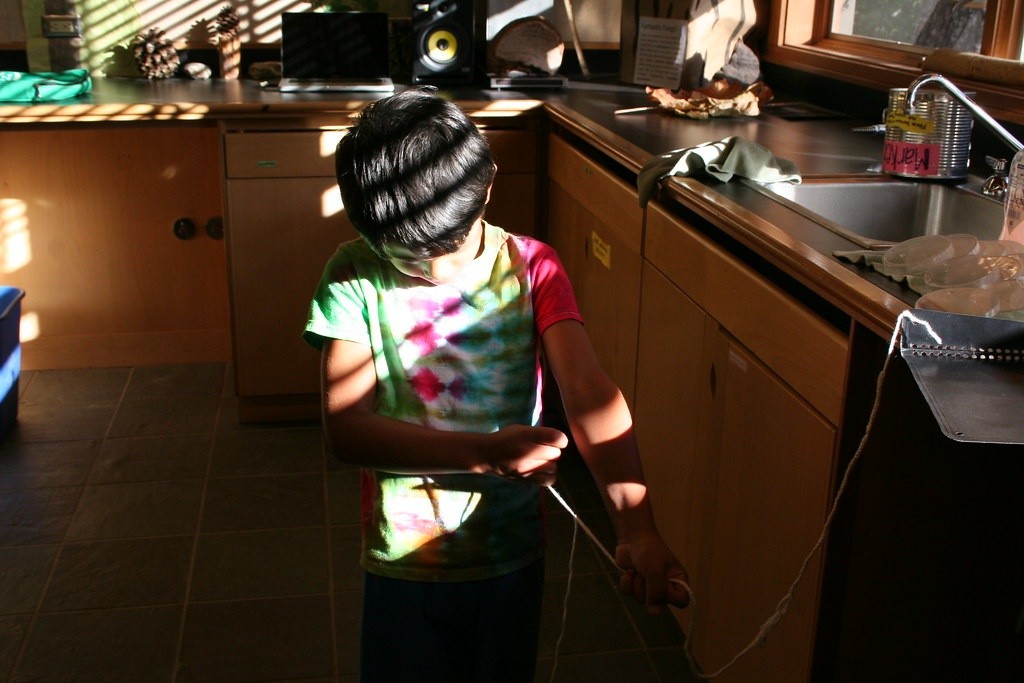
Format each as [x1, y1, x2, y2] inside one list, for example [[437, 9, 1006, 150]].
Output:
[[741, 172, 1003, 248]]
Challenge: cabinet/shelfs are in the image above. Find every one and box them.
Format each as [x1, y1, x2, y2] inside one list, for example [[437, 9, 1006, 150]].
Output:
[[0, 67, 1024, 683]]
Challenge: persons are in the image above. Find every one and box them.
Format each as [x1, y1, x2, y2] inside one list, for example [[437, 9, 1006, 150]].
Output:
[[303, 92, 690, 683]]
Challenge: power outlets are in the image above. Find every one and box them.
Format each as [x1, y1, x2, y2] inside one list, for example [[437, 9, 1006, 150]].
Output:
[[40, 13, 81, 38]]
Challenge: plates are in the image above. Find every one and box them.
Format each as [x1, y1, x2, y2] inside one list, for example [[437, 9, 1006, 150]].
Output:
[[763, 102, 835, 121]]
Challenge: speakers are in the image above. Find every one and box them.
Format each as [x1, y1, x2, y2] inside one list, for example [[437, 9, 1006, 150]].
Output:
[[410, 0, 489, 90]]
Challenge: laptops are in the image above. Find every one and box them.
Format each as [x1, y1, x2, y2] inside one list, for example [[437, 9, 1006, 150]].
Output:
[[279, 12, 395, 92]]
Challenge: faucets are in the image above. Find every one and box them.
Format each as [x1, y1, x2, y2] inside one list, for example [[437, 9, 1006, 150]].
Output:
[[905, 71, 1024, 151]]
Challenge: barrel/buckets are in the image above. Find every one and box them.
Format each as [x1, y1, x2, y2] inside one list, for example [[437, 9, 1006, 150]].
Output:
[[0, 286, 25, 455]]
